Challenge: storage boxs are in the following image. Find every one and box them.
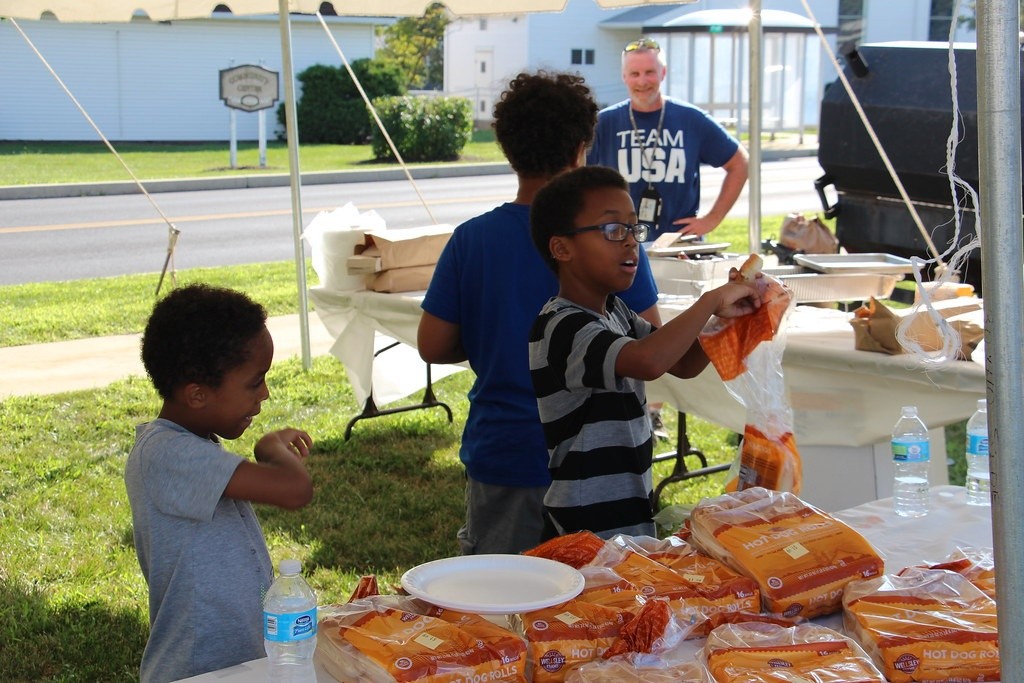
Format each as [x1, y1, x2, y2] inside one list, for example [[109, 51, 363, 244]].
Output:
[[639, 243, 899, 305]]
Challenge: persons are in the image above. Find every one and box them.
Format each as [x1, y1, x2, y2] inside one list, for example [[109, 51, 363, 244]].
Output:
[[415, 69, 664, 556], [525, 163, 761, 545], [123, 281, 319, 681], [575, 37, 749, 446]]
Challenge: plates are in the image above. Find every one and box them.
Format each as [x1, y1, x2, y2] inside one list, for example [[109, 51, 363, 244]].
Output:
[[401, 553, 585, 612]]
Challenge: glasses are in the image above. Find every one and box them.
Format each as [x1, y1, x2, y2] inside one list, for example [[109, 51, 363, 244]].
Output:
[[557, 223, 649, 243], [624, 38, 659, 52]]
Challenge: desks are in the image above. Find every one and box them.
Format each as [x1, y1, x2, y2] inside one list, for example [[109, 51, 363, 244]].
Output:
[[305, 284, 986, 513], [172, 483, 1003, 683]]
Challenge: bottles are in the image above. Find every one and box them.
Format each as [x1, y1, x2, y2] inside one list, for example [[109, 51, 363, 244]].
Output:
[[891, 406, 930, 518], [263, 558, 319, 683], [964, 398, 992, 507]]
